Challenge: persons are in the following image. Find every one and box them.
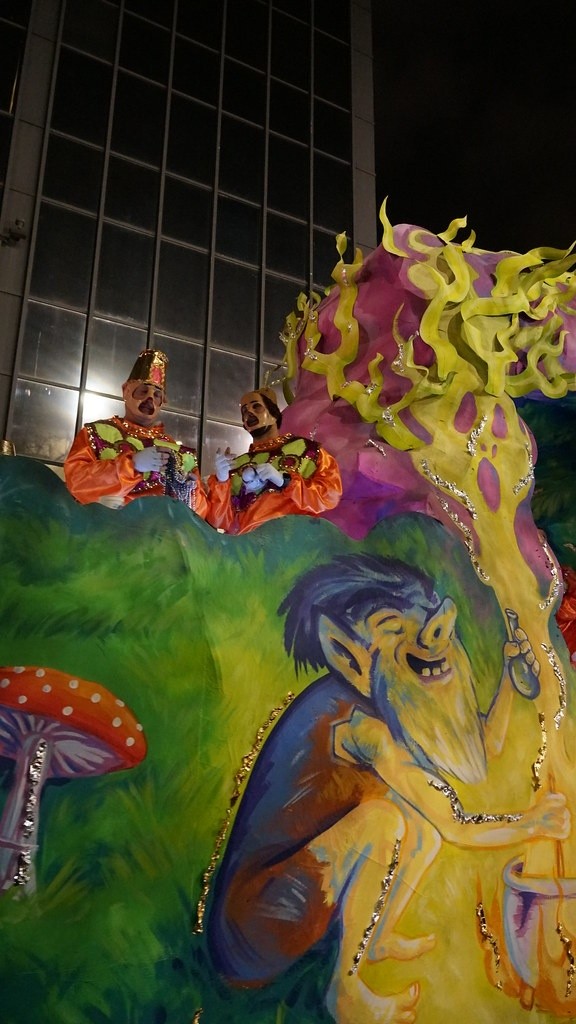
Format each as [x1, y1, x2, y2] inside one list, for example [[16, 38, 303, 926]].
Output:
[[206, 387, 342, 534], [63, 347, 210, 520]]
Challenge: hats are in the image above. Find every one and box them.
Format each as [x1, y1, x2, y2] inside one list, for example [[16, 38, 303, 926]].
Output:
[[253, 387, 277, 404], [127, 349, 169, 394]]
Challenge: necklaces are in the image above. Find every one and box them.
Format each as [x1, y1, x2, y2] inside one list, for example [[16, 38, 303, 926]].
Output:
[[159, 455, 196, 510]]
